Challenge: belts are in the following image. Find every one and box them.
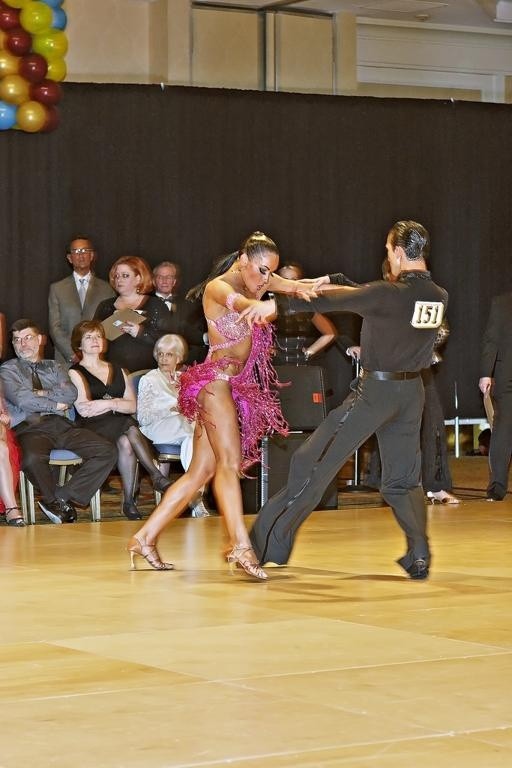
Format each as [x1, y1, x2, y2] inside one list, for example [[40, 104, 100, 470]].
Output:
[[367, 370, 418, 380]]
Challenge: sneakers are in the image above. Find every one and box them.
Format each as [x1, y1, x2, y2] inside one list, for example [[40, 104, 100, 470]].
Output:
[[37, 499, 67, 524], [410, 559, 427, 578], [64, 502, 77, 523]]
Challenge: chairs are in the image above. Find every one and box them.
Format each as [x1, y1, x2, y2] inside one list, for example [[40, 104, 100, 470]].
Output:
[[16, 369, 185, 523]]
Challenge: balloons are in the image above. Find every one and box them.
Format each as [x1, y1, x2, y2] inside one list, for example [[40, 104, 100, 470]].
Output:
[[0, 0, 69, 135]]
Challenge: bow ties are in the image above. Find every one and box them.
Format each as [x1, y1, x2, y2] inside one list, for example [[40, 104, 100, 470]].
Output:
[[160, 296, 174, 303]]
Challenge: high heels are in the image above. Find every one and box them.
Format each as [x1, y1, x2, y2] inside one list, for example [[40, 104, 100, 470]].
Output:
[[427, 490, 459, 504], [191, 501, 211, 518], [4, 507, 25, 527], [226, 545, 268, 580], [127, 533, 175, 570]]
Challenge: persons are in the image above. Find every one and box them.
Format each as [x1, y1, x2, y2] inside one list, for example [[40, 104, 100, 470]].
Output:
[[235, 219, 450, 581], [124, 227, 360, 583], [0, 234, 511, 526]]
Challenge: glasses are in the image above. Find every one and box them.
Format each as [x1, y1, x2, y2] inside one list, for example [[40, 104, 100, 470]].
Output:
[[71, 248, 93, 254], [14, 335, 35, 344]]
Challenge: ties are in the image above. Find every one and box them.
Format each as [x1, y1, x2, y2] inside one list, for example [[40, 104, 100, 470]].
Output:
[[32, 367, 42, 391]]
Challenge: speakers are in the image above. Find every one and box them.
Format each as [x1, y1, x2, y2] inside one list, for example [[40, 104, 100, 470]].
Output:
[[272, 366, 327, 431], [239, 434, 337, 514]]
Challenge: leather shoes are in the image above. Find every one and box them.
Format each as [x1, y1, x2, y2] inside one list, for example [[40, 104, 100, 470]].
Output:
[[487, 490, 503, 501], [152, 475, 175, 492], [123, 502, 142, 520]]
